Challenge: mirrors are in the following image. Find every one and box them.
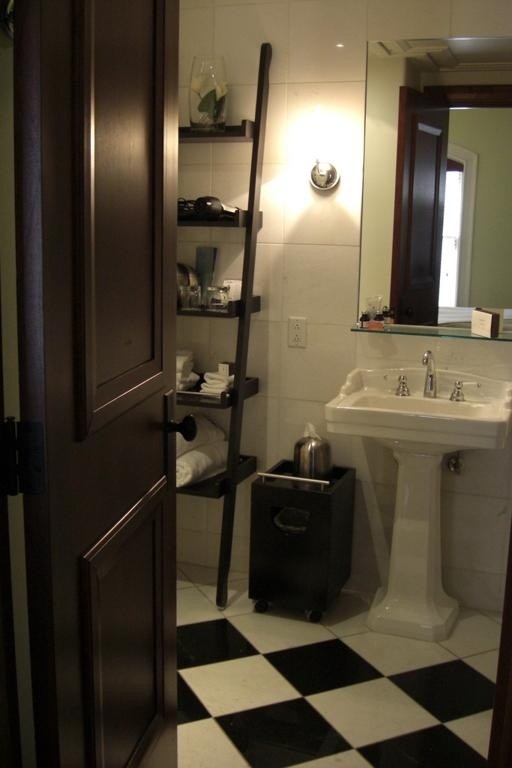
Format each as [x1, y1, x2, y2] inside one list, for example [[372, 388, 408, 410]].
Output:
[[352, 37, 512, 342]]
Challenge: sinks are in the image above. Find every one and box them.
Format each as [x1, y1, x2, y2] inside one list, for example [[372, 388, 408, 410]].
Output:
[[325, 368, 511, 450]]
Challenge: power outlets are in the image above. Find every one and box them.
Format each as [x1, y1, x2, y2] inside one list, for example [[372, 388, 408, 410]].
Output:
[[288, 316, 306, 349]]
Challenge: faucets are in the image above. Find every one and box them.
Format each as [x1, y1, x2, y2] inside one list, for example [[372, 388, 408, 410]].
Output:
[[420, 349, 438, 398]]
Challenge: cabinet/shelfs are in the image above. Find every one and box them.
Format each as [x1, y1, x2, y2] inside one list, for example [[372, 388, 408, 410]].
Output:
[[175, 42, 275, 608]]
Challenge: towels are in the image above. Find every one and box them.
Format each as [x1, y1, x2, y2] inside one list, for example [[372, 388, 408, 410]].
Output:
[[173, 418, 229, 488]]
